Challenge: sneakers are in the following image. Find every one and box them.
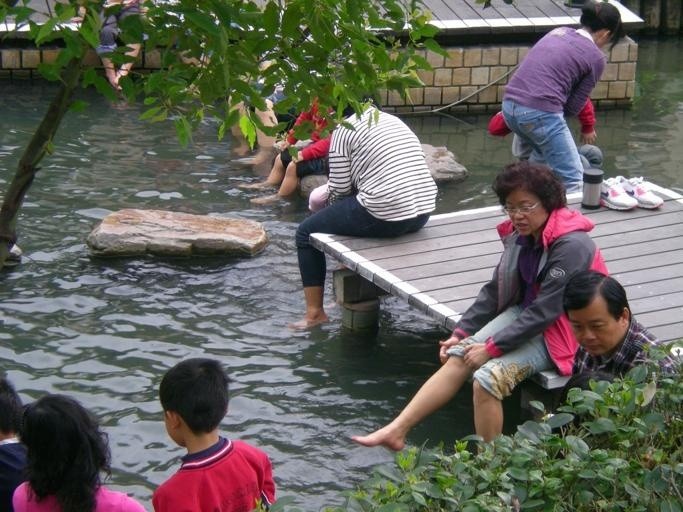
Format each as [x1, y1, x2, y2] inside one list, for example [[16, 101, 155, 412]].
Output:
[[600, 176, 664, 210]]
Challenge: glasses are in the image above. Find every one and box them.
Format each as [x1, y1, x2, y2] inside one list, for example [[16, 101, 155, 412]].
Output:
[[501, 202, 541, 215]]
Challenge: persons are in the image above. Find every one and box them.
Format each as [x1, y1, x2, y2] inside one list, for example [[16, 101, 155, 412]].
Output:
[[546, 369, 626, 458], [561, 271, 675, 384], [488, 88, 605, 173], [151, 356, 277, 512], [173, 30, 359, 208], [0, 376, 32, 511], [78, 2, 149, 102], [285, 92, 440, 332], [501, 1, 623, 192], [12, 393, 146, 511], [348, 159, 608, 510]]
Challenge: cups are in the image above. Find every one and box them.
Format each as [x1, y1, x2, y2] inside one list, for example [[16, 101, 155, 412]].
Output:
[[579, 168, 604, 210]]
